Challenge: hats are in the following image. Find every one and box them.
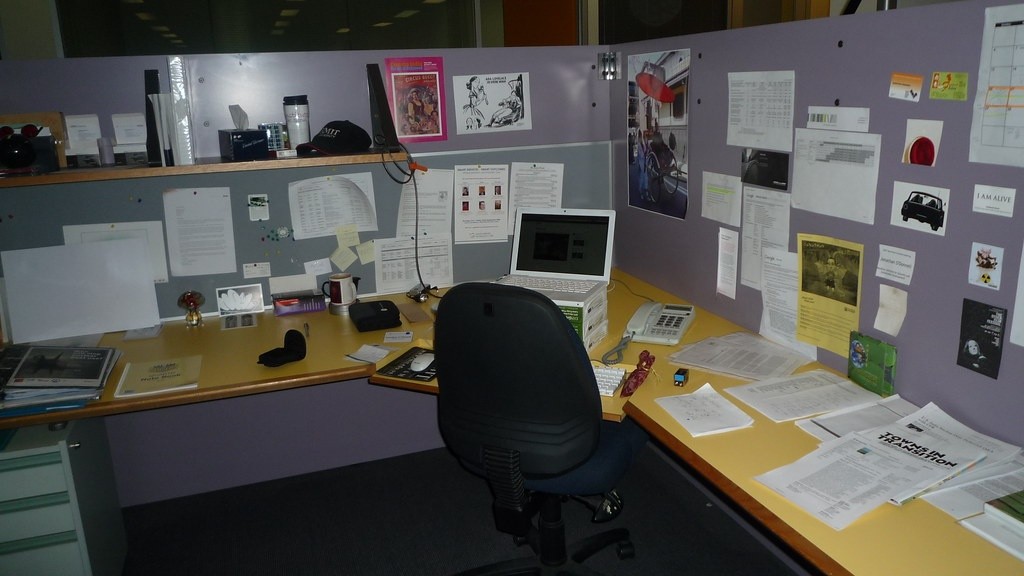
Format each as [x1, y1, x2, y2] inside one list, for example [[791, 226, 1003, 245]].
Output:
[[296, 120, 372, 156]]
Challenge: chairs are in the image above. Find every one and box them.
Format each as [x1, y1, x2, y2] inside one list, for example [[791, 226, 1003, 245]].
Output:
[[436, 283, 647, 576]]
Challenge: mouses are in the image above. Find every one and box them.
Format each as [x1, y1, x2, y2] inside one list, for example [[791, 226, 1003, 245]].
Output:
[[410, 353, 435, 372]]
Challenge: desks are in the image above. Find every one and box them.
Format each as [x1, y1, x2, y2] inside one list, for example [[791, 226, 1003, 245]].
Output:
[[0, 0, 1023, 576]]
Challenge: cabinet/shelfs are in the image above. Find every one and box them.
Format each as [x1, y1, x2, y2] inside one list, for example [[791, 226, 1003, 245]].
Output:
[[0, 417, 126, 576]]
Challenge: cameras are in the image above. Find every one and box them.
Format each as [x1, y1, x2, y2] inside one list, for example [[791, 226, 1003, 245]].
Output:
[[408, 284, 429, 303]]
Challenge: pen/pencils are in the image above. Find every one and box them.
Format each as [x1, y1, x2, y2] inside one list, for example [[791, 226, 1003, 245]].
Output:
[[304, 322, 309, 337]]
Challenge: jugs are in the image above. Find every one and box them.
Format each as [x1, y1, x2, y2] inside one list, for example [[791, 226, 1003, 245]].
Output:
[[322, 277, 361, 300]]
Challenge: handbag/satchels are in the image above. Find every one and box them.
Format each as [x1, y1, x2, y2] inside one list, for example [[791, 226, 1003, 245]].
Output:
[[349, 300, 403, 332]]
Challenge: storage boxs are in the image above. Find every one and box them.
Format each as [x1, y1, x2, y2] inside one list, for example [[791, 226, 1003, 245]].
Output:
[[0, 112, 67, 168], [271, 289, 326, 315], [493, 274, 607, 348]]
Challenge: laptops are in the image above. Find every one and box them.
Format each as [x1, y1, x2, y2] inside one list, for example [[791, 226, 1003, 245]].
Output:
[[497, 208, 617, 307]]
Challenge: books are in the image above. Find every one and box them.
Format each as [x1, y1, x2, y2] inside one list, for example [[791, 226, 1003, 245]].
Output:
[[0, 342, 122, 419], [114, 355, 202, 398], [984, 490, 1024, 539]]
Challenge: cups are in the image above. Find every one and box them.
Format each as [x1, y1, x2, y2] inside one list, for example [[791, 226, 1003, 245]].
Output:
[[329, 273, 357, 305]]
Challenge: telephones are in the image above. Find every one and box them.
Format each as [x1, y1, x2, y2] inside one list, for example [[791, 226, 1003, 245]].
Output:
[[620, 301, 696, 345]]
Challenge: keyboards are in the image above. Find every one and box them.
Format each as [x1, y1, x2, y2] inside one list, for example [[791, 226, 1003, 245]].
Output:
[[592, 364, 627, 397]]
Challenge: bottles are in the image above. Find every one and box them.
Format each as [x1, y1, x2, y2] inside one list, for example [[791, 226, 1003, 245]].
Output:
[[282, 94, 311, 150]]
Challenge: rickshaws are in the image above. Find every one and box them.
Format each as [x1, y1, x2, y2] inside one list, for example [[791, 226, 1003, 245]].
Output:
[[639, 129, 687, 203]]
